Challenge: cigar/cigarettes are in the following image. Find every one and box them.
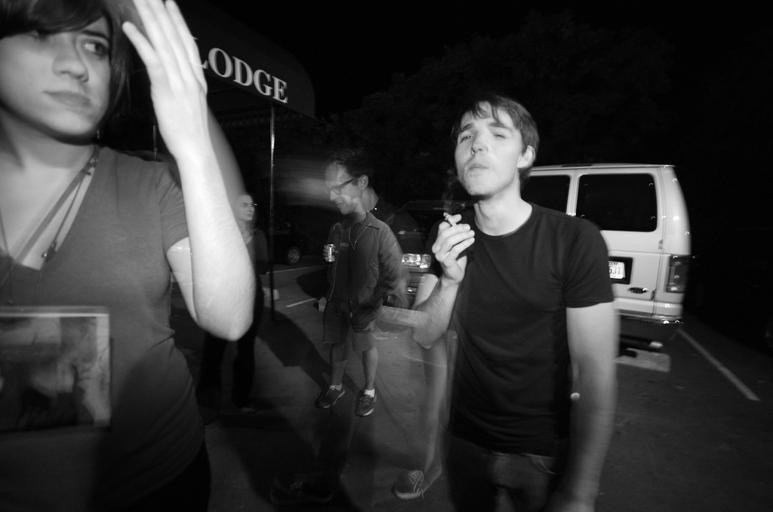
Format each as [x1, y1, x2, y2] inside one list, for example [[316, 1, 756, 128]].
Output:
[[442, 212, 454, 226]]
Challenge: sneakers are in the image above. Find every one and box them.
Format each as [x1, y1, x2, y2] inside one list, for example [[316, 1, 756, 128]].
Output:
[[317, 388, 346, 408], [357, 393, 377, 416], [394, 460, 442, 500]]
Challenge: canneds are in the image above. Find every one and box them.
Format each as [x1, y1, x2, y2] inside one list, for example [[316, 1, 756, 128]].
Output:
[[326, 244, 338, 261]]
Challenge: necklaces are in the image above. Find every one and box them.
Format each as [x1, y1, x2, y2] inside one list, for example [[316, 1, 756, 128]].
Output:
[[1, 161, 93, 305]]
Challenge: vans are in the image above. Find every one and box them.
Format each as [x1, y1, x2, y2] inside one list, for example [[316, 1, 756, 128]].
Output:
[[520, 162, 690, 350]]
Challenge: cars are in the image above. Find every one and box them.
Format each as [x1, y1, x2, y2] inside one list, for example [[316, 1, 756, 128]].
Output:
[[381, 200, 472, 295]]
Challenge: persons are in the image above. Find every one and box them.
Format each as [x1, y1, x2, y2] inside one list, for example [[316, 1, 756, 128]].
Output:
[[316, 153, 424, 253], [394, 244, 450, 498], [230, 193, 262, 403], [409, 89, 620, 512], [313, 185, 402, 415], [0, 0, 256, 512]]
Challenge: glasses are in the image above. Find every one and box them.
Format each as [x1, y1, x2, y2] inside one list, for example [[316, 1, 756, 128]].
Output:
[[325, 179, 353, 195]]
